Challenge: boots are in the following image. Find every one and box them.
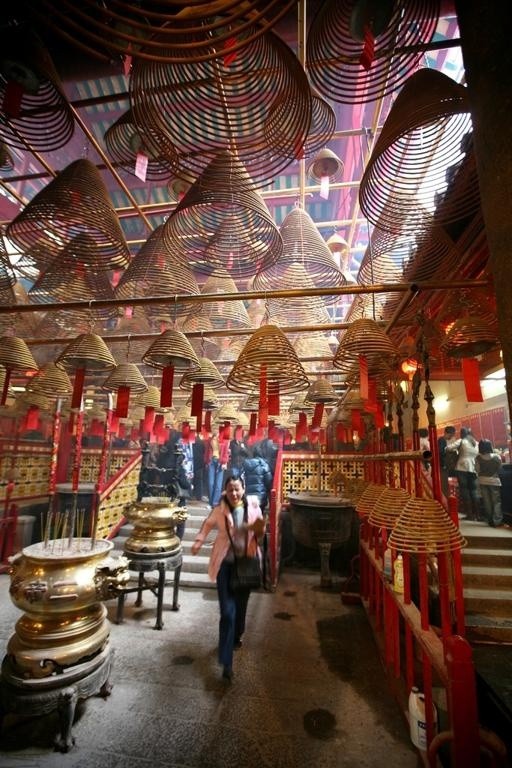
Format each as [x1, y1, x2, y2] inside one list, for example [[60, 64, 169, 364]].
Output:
[[462, 499, 474, 520], [474, 501, 483, 521]]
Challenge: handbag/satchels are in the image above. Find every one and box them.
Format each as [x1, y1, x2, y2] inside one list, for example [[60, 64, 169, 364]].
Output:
[[446, 451, 458, 471], [229, 558, 262, 592], [174, 482, 191, 499]]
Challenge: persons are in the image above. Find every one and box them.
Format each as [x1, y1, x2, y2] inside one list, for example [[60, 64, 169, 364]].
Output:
[[439, 426, 456, 497], [474, 439, 504, 528], [192, 476, 267, 684], [192, 424, 279, 512], [444, 427, 485, 522], [419, 428, 430, 452], [48, 431, 148, 447], [156, 429, 195, 540]]
